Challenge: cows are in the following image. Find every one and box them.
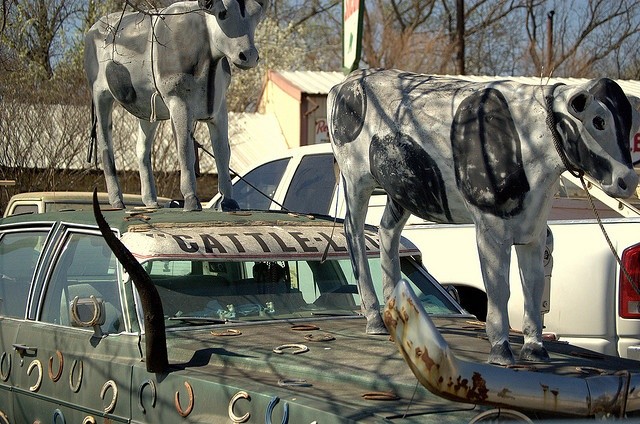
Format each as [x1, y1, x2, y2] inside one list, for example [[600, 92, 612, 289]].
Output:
[[326, 66, 640, 367], [82, 0, 269, 213]]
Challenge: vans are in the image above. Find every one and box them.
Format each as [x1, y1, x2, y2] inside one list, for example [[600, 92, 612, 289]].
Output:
[[4, 192, 180, 218]]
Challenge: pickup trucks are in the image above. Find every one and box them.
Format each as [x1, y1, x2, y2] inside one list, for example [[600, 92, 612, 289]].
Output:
[[0, 206, 640, 423], [203, 144, 639, 363]]
